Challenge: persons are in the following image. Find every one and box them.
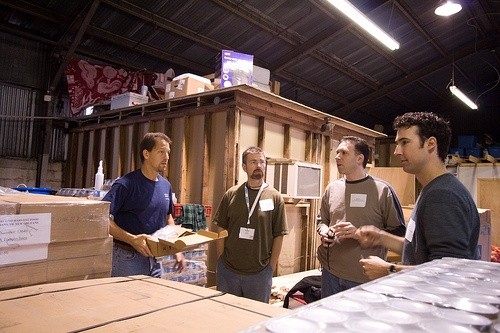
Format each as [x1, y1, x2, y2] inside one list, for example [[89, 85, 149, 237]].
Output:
[[211, 147, 288, 305], [316, 137, 405, 301], [355, 112, 480, 281], [99, 132, 186, 277]]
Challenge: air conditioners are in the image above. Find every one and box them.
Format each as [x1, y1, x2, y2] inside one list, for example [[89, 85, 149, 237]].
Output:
[[263, 160, 322, 199]]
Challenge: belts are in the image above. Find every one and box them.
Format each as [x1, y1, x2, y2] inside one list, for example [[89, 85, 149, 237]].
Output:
[[113, 242, 136, 251]]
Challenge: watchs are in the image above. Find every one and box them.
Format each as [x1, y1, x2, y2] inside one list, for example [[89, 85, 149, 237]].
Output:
[[387, 264, 397, 274]]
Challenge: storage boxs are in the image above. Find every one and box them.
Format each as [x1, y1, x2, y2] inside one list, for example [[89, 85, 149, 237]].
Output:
[[85, 103, 111, 116], [1, 193, 292, 333], [402, 202, 492, 262], [111, 92, 148, 110], [165, 49, 272, 98]]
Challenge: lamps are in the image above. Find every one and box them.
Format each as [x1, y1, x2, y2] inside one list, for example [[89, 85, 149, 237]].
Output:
[[434, 0, 463, 17], [320, 116, 336, 133], [327, 0, 401, 50], [449, 63, 480, 110]]
